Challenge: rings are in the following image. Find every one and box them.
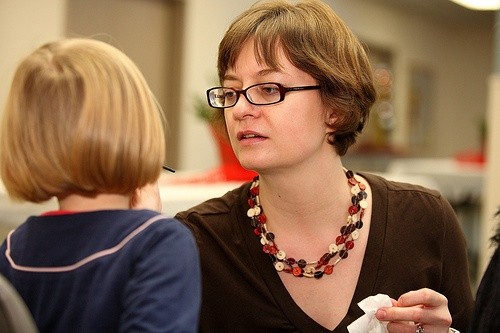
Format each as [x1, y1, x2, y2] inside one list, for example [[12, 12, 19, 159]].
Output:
[[414, 321, 426, 333]]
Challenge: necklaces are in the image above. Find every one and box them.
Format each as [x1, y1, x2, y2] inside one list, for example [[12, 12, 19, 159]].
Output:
[[247, 166, 368, 279]]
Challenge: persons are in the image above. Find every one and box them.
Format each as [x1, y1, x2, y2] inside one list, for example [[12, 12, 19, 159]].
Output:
[[474, 204, 500, 333], [172, 0, 480, 333], [0, 38, 201, 333]]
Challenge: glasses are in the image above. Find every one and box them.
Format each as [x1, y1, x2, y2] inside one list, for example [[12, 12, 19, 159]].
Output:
[[207, 82, 323, 109]]
